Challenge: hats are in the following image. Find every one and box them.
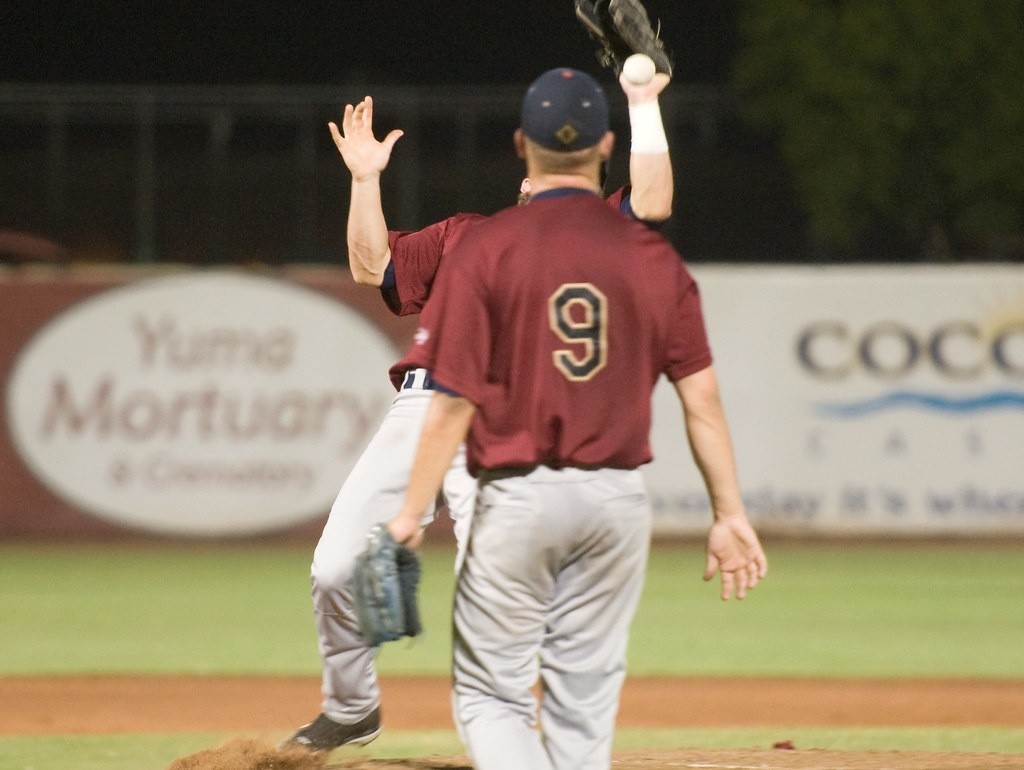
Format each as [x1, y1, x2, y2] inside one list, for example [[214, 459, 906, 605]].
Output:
[[522, 68, 609, 151]]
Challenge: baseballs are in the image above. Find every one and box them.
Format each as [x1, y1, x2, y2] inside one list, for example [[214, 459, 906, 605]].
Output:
[[622, 52, 657, 86]]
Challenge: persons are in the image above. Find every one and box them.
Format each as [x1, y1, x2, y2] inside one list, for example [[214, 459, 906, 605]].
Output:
[[383, 66, 776, 770], [280, 70, 675, 761]]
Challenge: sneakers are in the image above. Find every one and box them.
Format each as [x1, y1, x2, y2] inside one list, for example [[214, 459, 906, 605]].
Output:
[[289, 708, 382, 753]]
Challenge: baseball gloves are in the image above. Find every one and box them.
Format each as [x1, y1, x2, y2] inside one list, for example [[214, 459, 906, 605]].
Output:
[[574, 0, 672, 78], [353, 520, 423, 648]]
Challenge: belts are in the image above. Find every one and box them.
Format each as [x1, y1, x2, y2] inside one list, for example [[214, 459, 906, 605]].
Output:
[[403, 374, 429, 389]]
